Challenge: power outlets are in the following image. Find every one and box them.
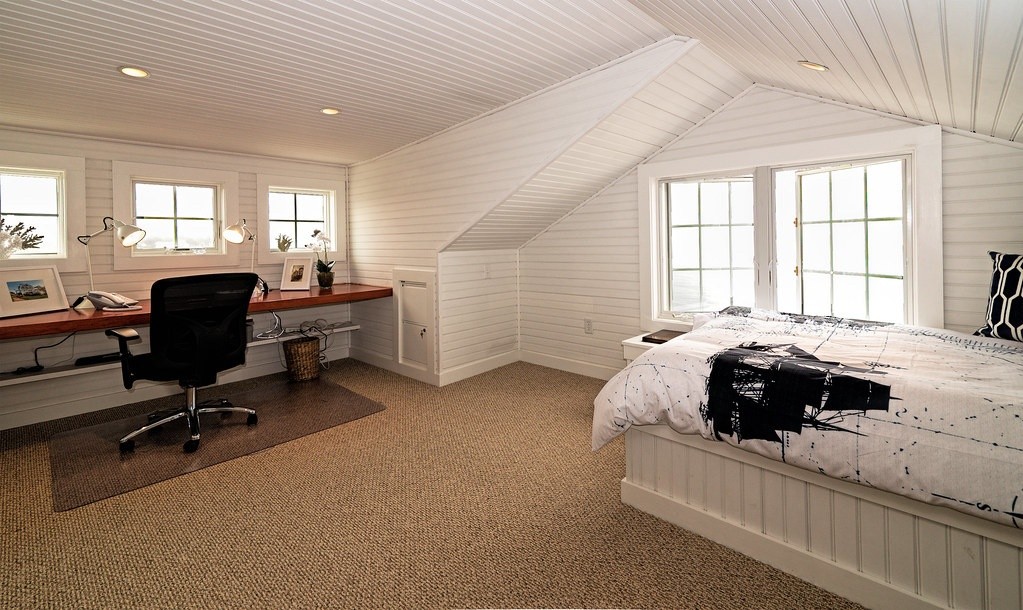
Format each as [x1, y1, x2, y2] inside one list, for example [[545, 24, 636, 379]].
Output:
[[584, 319, 594, 334]]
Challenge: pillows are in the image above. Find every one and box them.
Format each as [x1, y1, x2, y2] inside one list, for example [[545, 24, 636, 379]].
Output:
[[973, 251, 1023, 343]]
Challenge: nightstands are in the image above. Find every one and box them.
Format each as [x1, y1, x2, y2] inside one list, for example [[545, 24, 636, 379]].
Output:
[[620, 333, 660, 368]]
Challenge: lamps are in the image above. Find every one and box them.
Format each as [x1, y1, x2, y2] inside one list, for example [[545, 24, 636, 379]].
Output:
[[222, 220, 256, 274], [76, 217, 147, 291]]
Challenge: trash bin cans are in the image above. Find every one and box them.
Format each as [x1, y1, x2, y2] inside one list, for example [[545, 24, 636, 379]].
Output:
[[283, 337, 319, 382]]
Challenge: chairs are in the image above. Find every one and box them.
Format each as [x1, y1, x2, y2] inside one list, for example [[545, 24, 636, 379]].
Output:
[[106, 271, 259, 456]]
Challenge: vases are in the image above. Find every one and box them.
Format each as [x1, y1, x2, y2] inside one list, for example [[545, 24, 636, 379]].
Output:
[[316, 271, 334, 289]]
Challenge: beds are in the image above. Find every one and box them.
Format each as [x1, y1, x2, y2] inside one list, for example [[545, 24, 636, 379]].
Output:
[[591, 307, 1023, 610]]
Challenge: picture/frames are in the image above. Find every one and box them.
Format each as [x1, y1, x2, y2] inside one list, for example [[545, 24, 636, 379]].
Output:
[[0, 265, 70, 319], [279, 257, 312, 291]]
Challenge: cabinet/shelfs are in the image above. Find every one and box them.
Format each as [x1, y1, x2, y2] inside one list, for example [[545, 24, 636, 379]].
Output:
[[0, 281, 392, 388]]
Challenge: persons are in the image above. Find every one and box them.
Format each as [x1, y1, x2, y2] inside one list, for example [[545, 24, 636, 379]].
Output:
[[291, 268, 303, 282]]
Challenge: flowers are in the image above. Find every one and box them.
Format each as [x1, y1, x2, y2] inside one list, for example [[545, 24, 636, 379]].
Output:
[[308, 230, 335, 273]]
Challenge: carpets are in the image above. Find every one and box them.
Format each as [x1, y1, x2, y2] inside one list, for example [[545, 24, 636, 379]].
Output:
[[49, 376, 389, 510]]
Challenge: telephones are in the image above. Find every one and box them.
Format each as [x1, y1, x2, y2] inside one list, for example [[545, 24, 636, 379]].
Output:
[[86, 291, 137, 310]]
